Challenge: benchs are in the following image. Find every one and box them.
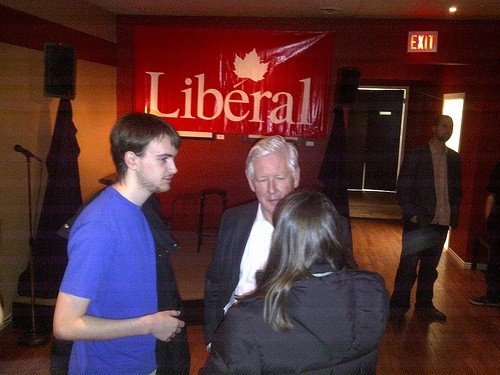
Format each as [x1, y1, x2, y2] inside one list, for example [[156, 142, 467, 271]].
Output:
[[473, 233, 491, 271]]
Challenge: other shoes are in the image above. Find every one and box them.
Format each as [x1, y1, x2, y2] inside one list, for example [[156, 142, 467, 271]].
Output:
[[414, 303, 446, 320], [469, 294, 500, 306], [390, 306, 406, 324]]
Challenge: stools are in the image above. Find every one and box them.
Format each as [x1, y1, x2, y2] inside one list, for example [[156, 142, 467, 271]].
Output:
[[196, 188, 227, 252]]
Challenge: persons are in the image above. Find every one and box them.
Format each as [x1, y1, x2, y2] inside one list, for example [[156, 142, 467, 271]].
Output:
[[198, 187, 390, 375], [389, 115, 462, 324], [203, 137, 352, 352], [469, 161, 500, 307], [53, 112, 186, 375]]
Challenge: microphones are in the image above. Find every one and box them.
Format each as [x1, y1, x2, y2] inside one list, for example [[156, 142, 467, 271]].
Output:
[[14, 144, 44, 162]]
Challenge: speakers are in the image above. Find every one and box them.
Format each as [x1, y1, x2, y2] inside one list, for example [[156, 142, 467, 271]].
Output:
[[333, 67, 362, 115], [45, 43, 77, 100]]
